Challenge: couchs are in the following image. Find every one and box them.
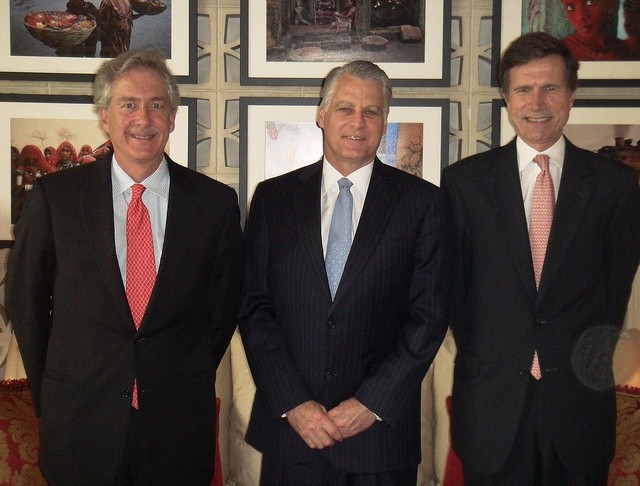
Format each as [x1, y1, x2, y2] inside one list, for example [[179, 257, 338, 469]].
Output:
[[2, 316, 640, 486], [1, 378, 223, 483], [439, 379, 640, 481]]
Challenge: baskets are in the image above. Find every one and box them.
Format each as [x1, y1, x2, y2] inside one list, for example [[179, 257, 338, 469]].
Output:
[[132, 0, 167, 14]]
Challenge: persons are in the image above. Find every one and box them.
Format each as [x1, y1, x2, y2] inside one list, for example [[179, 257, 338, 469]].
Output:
[[437, 31, 640, 486], [3, 47, 248, 485], [230, 59, 454, 486], [44, 146, 56, 161], [11, 145, 20, 214], [80, 155, 97, 165], [94, 147, 111, 160], [328, 0, 357, 32], [559, 0, 633, 60], [48, 140, 81, 172], [622, 0, 640, 60], [77, 143, 93, 158], [293, 0, 311, 25], [15, 144, 56, 224], [90, 0, 152, 58]]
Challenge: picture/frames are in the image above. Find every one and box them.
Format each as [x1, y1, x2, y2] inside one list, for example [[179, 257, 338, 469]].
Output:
[[0, 94, 196, 245], [241, 96, 413, 234], [239, 2, 451, 88], [2, 1, 198, 86], [490, 2, 639, 89]]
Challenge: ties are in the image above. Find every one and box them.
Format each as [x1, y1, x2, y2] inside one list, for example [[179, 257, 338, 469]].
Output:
[[528, 154, 555, 381], [125, 184, 158, 410], [324, 177, 354, 303]]
